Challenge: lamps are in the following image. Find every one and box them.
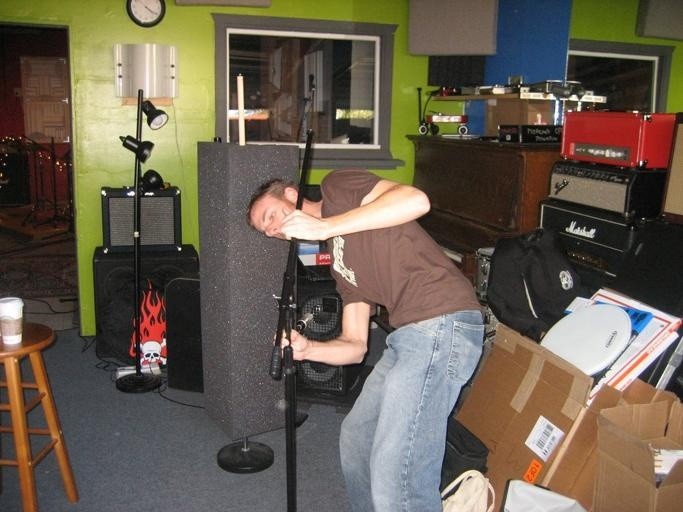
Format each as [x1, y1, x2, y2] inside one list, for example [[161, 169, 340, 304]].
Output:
[[115, 89, 170, 393]]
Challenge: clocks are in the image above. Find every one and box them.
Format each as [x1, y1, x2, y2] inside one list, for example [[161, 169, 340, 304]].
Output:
[[125, 0, 164, 28]]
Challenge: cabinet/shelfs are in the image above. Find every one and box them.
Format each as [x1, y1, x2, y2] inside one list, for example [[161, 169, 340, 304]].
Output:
[[405, 134, 562, 258]]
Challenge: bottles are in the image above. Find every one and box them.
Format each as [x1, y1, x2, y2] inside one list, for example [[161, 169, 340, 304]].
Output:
[[0, 296, 25, 344]]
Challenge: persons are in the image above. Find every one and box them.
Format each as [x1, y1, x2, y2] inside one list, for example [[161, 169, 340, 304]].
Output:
[[244, 166, 486, 512]]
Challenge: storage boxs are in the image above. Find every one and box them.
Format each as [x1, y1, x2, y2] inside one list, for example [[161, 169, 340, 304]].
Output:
[[559, 109, 677, 169]]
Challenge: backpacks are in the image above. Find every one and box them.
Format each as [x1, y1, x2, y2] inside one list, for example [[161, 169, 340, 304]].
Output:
[[482, 221, 580, 334]]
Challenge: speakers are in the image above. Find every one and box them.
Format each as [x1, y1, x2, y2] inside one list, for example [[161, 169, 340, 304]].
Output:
[[287, 265, 368, 412], [165, 274, 203, 393], [93, 245, 200, 359]]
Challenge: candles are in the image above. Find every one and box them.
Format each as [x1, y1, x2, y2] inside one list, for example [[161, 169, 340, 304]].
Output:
[[236, 72, 247, 146]]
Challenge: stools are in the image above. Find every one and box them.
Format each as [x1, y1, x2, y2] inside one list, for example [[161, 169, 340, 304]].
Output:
[[0, 322, 79, 512]]
